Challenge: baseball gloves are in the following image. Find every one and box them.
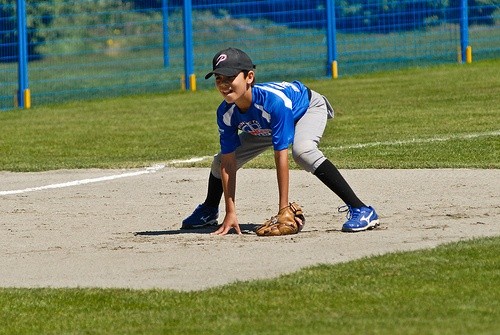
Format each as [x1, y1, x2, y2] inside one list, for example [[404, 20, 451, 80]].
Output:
[[256, 202, 306, 236]]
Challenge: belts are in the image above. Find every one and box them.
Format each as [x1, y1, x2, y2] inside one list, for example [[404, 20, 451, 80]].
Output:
[[294, 84, 312, 125]]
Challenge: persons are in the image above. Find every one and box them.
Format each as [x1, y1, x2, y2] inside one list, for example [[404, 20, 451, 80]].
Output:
[[183, 47, 380, 236]]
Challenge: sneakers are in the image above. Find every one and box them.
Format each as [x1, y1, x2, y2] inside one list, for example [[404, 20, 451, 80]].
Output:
[[182, 204, 219, 228], [338, 205, 380, 231]]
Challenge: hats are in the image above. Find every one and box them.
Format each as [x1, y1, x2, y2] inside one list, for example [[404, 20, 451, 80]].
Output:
[[205, 48, 256, 79]]
